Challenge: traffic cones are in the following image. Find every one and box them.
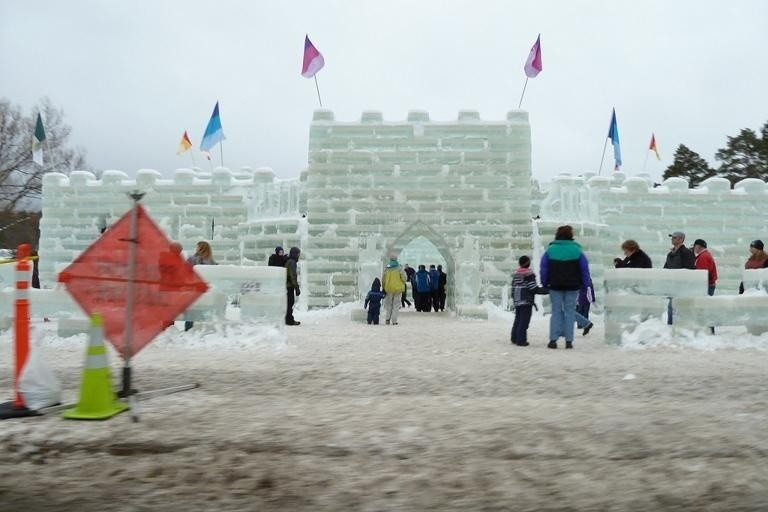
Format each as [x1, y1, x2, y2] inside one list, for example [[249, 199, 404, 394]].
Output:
[[1, 246, 59, 420]]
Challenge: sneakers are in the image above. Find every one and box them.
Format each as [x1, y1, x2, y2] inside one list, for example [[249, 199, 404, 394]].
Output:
[[515, 339, 531, 348], [581, 322, 595, 336], [563, 340, 575, 349], [547, 342, 556, 350], [288, 320, 301, 327]]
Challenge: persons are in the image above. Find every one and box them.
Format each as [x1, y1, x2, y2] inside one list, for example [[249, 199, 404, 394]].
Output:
[[689, 238, 718, 296], [157, 242, 183, 330], [663, 231, 696, 324], [404, 264, 416, 282], [412, 265, 433, 312], [285, 246, 301, 325], [364, 277, 386, 325], [540, 225, 592, 349], [268, 247, 288, 267], [401, 283, 411, 308], [576, 278, 595, 329], [411, 265, 420, 309], [559, 304, 594, 336], [30, 249, 40, 289], [429, 265, 440, 312], [739, 240, 768, 294], [613, 239, 652, 268], [185, 241, 218, 265], [511, 255, 550, 346], [437, 264, 447, 312], [382, 255, 408, 325]]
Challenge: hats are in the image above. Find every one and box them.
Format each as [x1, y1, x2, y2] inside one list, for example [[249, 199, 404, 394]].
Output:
[[273, 246, 284, 257], [666, 230, 685, 242], [689, 238, 708, 248], [389, 252, 398, 262], [750, 239, 764, 251]]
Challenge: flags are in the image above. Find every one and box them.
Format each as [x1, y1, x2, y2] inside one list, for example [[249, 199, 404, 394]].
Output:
[[607, 108, 622, 171], [301, 32, 325, 78], [523, 33, 542, 78], [176, 129, 192, 155], [32, 113, 46, 167], [649, 133, 661, 160], [199, 100, 227, 152]]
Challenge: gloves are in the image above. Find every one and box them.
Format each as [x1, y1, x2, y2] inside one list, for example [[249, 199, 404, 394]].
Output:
[[295, 288, 301, 296], [364, 304, 367, 309]]
